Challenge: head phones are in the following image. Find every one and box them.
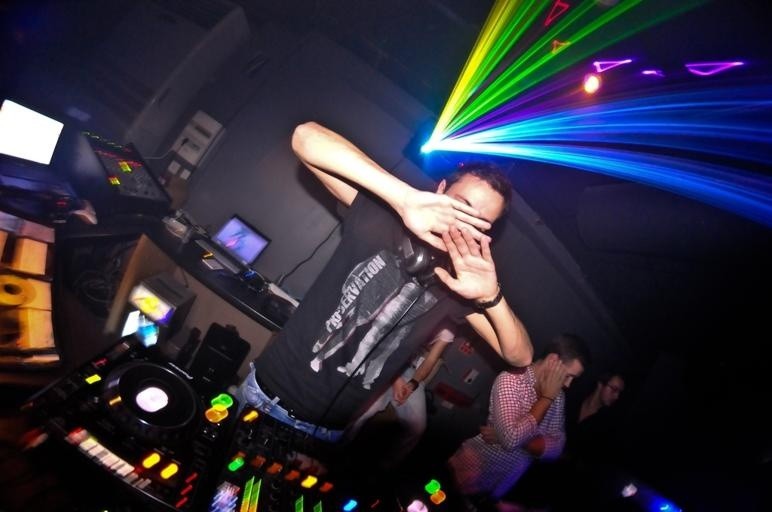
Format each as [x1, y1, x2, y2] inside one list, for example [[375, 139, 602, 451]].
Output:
[[244, 269, 267, 292], [399, 230, 448, 287]]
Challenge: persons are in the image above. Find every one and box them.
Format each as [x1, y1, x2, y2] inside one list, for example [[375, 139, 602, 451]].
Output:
[[448, 330, 591, 511], [234, 119, 536, 472], [349, 308, 468, 460], [503, 365, 627, 512]]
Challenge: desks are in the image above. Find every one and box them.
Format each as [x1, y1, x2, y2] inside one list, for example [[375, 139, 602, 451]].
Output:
[[101, 224, 298, 336]]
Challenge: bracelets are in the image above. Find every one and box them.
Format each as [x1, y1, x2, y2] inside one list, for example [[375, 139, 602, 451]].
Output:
[[479, 288, 506, 310], [409, 377, 424, 390], [539, 395, 555, 402]]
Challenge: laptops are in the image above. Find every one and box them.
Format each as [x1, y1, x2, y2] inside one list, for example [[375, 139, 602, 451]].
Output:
[[196, 212, 271, 274]]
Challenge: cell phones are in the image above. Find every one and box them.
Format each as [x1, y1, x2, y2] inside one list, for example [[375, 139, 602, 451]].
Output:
[[202, 258, 225, 271]]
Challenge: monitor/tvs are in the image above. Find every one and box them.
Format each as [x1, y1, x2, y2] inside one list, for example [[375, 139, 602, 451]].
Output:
[[0, 99, 65, 167]]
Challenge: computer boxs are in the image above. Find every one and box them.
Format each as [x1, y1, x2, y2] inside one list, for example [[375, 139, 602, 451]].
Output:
[[188, 322, 251, 400], [116, 270, 196, 363]]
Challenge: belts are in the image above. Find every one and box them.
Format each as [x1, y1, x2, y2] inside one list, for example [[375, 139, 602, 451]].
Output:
[[255, 371, 309, 422]]
